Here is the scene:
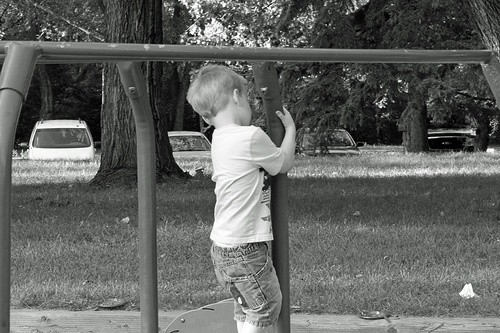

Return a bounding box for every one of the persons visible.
[186,63,296,332]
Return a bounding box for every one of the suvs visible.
[19,118,96,162]
[296,127,364,158]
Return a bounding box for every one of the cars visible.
[426,126,476,153]
[167,130,213,178]
